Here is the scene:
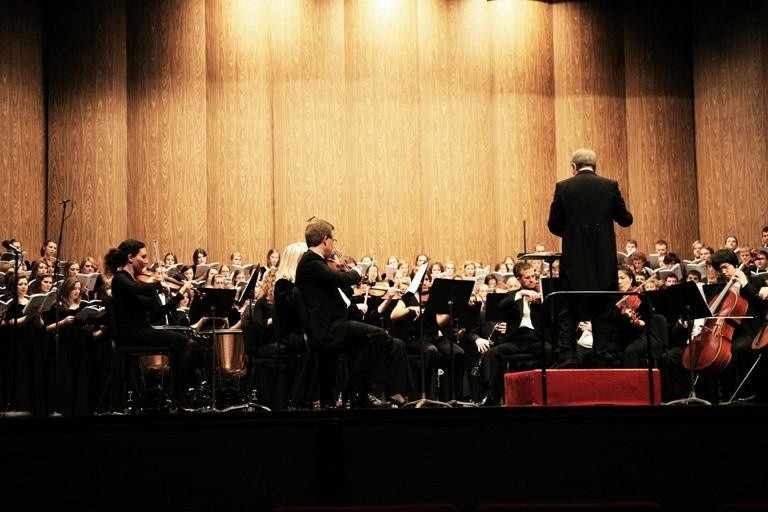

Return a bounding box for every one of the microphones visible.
[57,199,70,204]
[1,240,22,254]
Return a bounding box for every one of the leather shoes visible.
[737,395,760,403]
[477,397,492,406]
[368,393,391,408]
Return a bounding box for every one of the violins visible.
[137,266,201,296]
[352,281,405,297]
[615,274,640,325]
[327,259,375,285]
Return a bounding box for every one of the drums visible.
[216,329,247,378]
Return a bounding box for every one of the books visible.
[644,266,666,274]
[0,260,15,267]
[687,260,707,279]
[191,281,206,287]
[475,266,490,276]
[166,263,183,272]
[0,287,6,290]
[75,273,99,291]
[194,262,220,277]
[75,306,105,321]
[1,298,14,313]
[26,278,37,285]
[0,293,7,298]
[53,280,65,291]
[659,263,683,281]
[617,248,636,264]
[21,292,57,315]
[236,281,249,287]
[648,254,660,268]
[79,299,102,307]
[463,276,485,294]
[357,261,375,273]
[0,272,7,275]
[682,258,702,273]
[231,264,253,271]
[386,265,397,278]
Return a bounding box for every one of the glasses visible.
[331,238,337,245]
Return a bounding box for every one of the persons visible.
[232,270,246,286]
[32,273,54,294]
[724,235,741,264]
[257,247,280,282]
[691,240,705,260]
[201,267,218,287]
[162,252,177,267]
[430,262,443,274]
[463,261,476,277]
[453,275,463,280]
[365,264,381,282]
[189,248,210,281]
[8,239,23,254]
[631,252,654,276]
[62,259,80,279]
[702,249,759,403]
[534,242,545,254]
[484,275,498,288]
[228,252,242,271]
[628,265,636,274]
[758,286,768,300]
[28,260,48,281]
[409,254,428,281]
[617,266,655,369]
[46,277,107,419]
[686,270,701,283]
[547,148,633,369]
[506,276,521,292]
[739,244,752,265]
[469,284,512,406]
[761,226,768,248]
[482,262,561,406]
[38,240,60,261]
[347,287,408,409]
[390,268,466,406]
[172,273,188,281]
[211,274,225,289]
[656,240,668,267]
[634,273,645,286]
[445,261,456,276]
[392,270,403,283]
[360,255,372,263]
[664,274,678,287]
[254,269,279,329]
[495,263,509,273]
[700,257,726,284]
[398,261,408,276]
[504,256,515,273]
[527,259,548,278]
[219,264,231,277]
[254,280,260,301]
[626,239,637,253]
[754,251,768,269]
[104,238,194,412]
[663,252,680,270]
[43,256,55,273]
[182,266,194,280]
[0,275,45,412]
[700,247,715,283]
[177,291,191,309]
[274,243,309,335]
[295,219,394,407]
[80,256,105,300]
[644,279,659,291]
[381,256,399,287]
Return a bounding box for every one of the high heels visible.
[386,395,407,407]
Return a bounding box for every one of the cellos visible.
[682,249,759,402]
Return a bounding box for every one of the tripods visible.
[200,314,222,414]
[661,376,713,405]
[222,305,272,412]
[402,288,452,410]
[442,318,480,409]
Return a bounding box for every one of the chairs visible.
[100,295,176,412]
[288,286,359,412]
